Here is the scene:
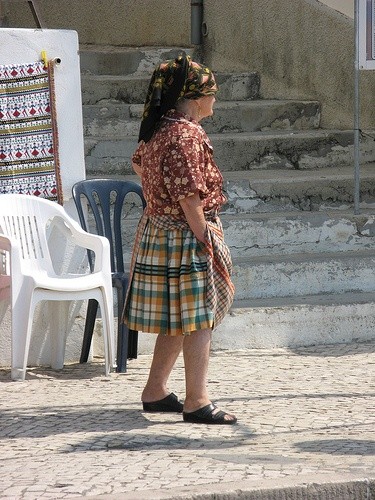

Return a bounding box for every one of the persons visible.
[120,56,238,425]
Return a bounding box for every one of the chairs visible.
[0,193,116,382]
[71,178,146,363]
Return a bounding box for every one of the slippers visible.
[182,400,238,426]
[141,392,185,415]
